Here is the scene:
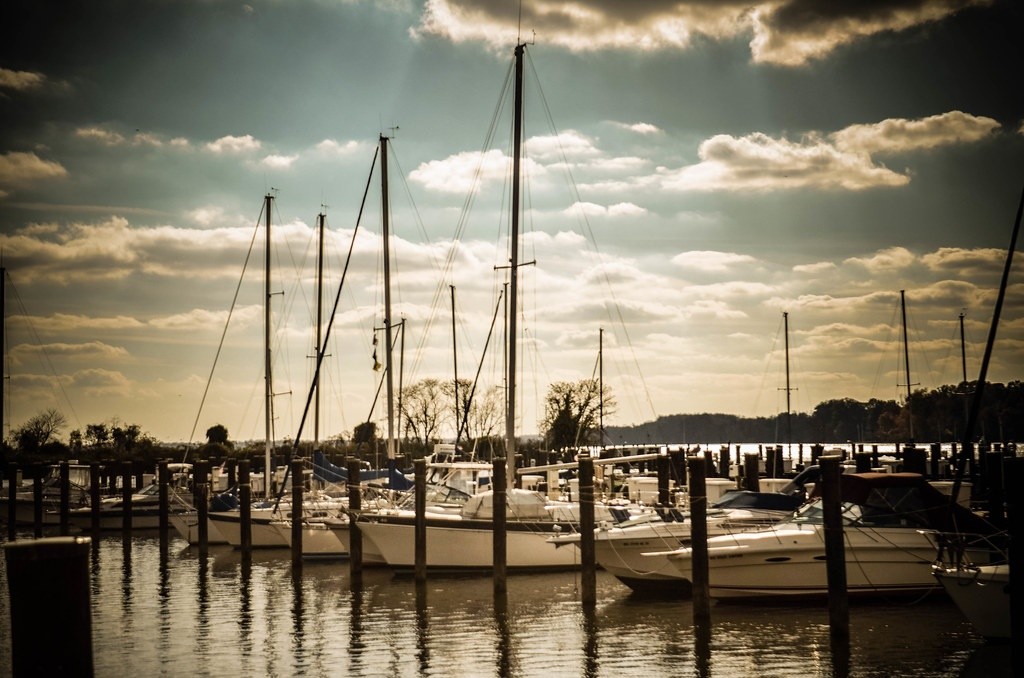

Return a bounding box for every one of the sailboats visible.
[0,0,1024,598]
